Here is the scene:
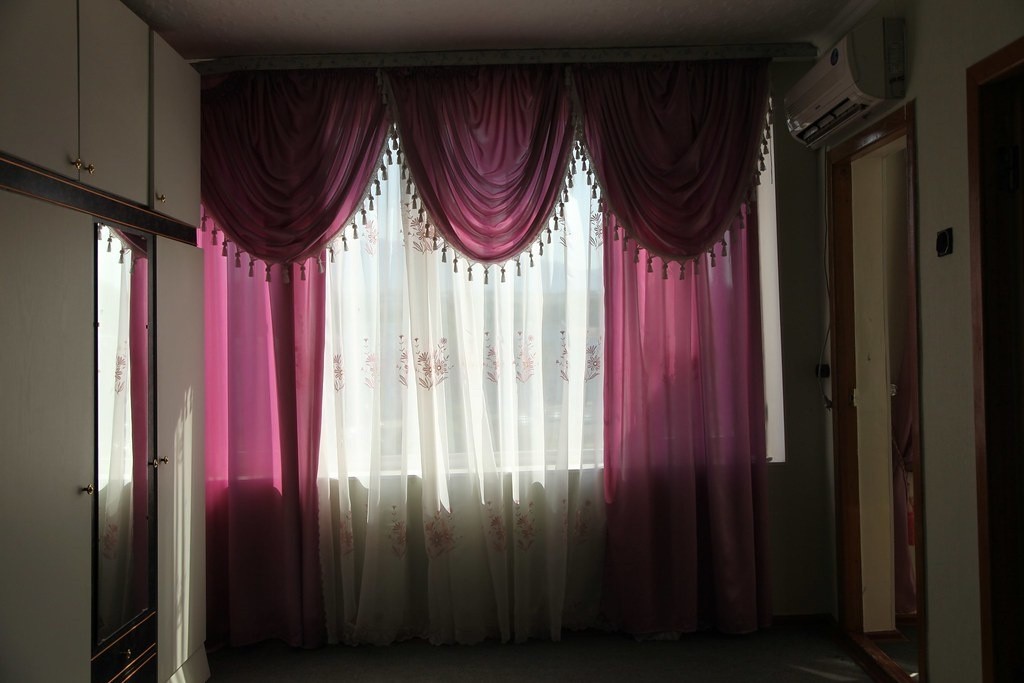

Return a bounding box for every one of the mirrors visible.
[92,221,158,646]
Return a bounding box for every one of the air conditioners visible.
[782,17,909,150]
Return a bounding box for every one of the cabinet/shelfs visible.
[90,609,159,683]
[147,29,203,228]
[0,185,93,682]
[0,0,148,205]
[151,230,206,681]
